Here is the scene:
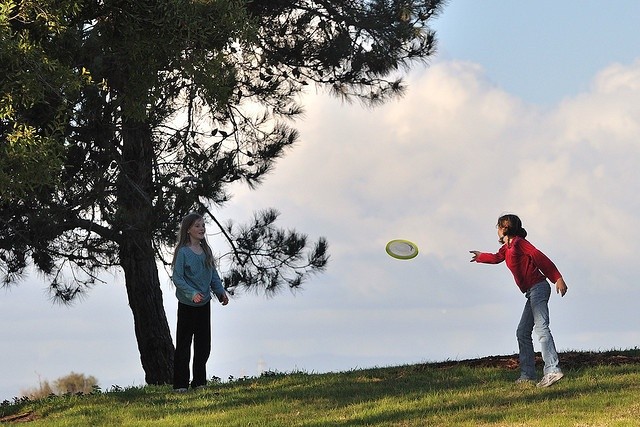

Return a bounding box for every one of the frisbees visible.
[386,239,419,259]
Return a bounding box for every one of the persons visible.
[170,214,229,394]
[469,214,568,388]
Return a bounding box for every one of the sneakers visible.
[514,378,529,383]
[536,368,564,387]
